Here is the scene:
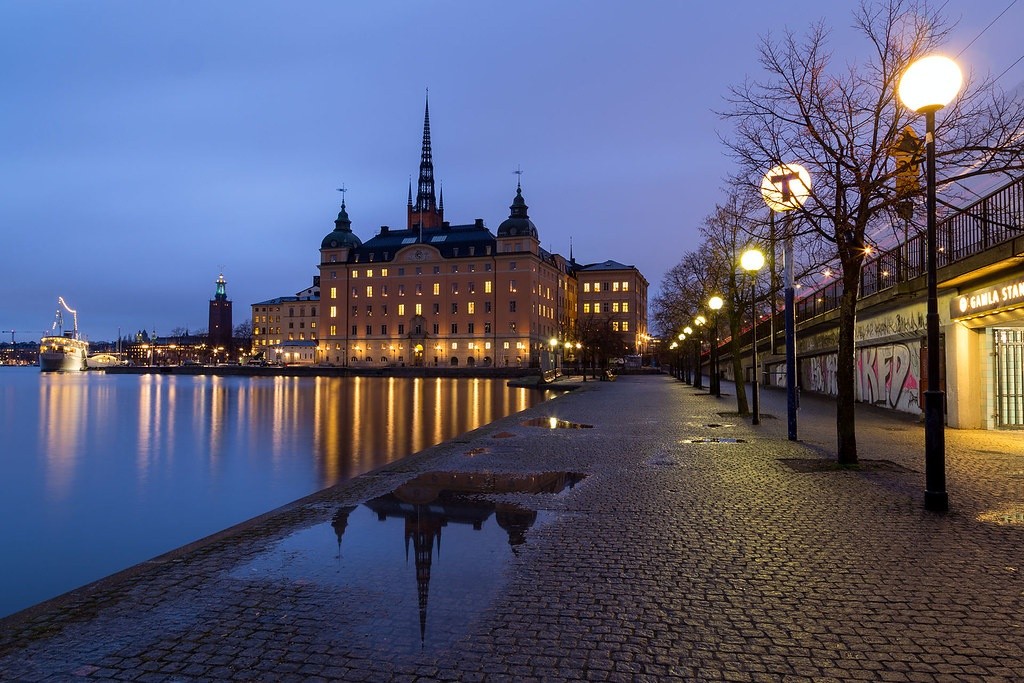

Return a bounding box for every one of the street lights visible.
[684,327,692,385]
[565,343,571,378]
[679,334,686,382]
[740,249,765,425]
[709,296,724,398]
[897,55,964,514]
[551,339,557,382]
[760,163,811,440]
[695,316,706,390]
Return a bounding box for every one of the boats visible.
[86,354,129,370]
[39,295,90,372]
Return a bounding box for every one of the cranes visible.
[1,329,45,346]
